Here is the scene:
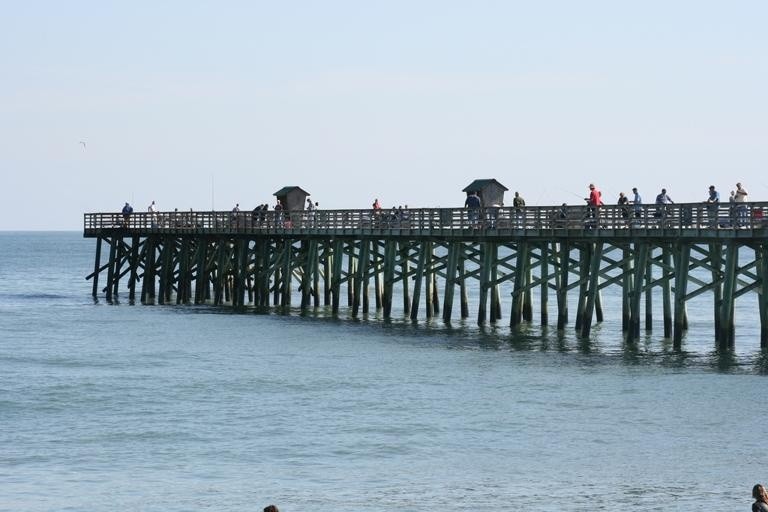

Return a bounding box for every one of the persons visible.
[752,484,768,512]
[463,182,748,229]
[372,198,410,227]
[121,198,320,228]
